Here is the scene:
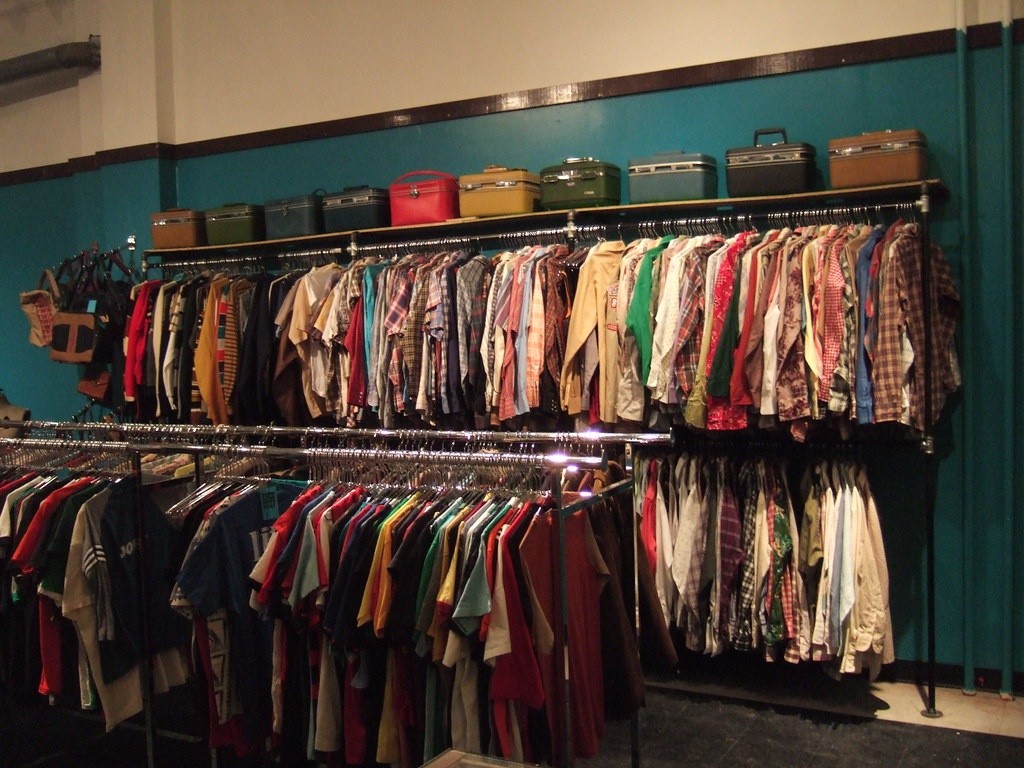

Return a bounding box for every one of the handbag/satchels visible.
[48,265,100,363]
[826,128,928,189]
[50,241,142,346]
[265,196,321,240]
[389,170,458,226]
[78,366,142,405]
[19,270,63,347]
[151,208,207,249]
[204,204,265,245]
[458,164,540,217]
[626,150,732,206]
[724,128,829,203]
[320,184,391,230]
[538,158,623,210]
